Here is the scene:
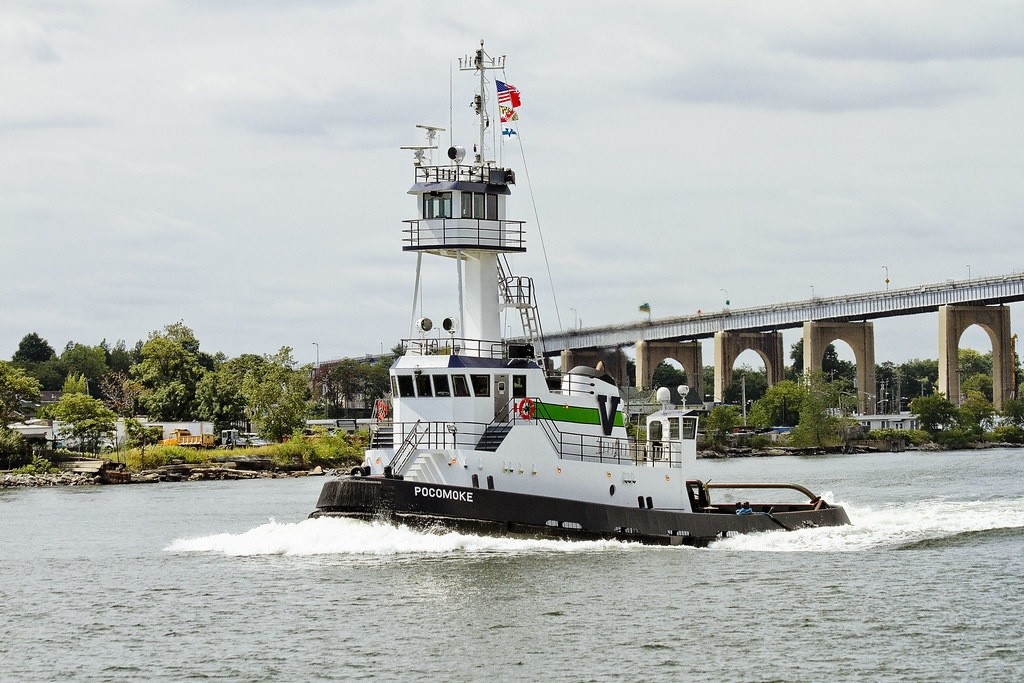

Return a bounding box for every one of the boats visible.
[307,39,853,551]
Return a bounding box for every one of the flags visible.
[640,304,649,311]
[496,79,518,92]
[499,105,518,122]
[501,125,518,138]
[497,91,521,108]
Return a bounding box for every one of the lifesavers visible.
[377,401,388,420]
[519,398,535,420]
